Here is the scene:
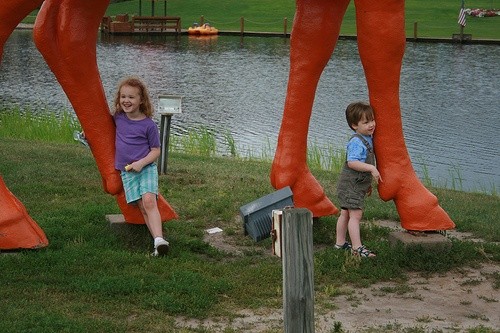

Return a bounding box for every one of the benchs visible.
[131,15,181,32]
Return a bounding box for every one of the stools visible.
[101,13,129,28]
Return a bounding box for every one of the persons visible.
[81,78,170,256]
[335,101,383,257]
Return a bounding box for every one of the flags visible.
[457,0,466,27]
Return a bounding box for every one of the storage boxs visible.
[110,22,134,33]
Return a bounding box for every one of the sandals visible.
[334,242,351,251]
[351,245,376,258]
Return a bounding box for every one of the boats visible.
[188,23,219,35]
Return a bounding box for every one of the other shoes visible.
[154,237,169,256]
